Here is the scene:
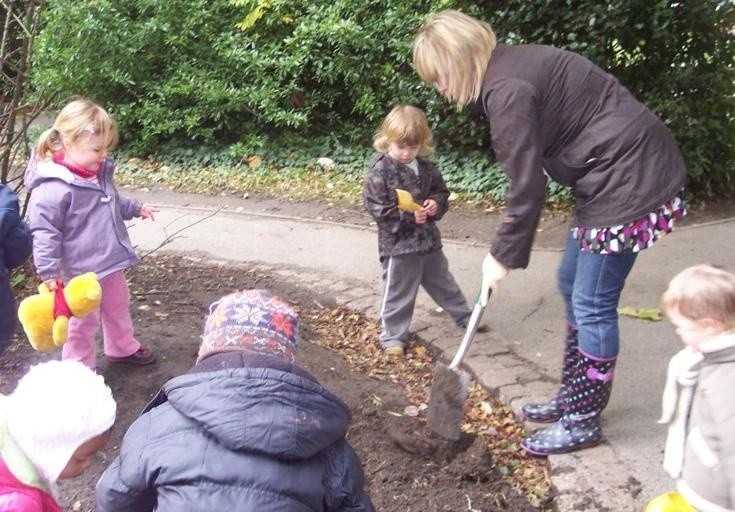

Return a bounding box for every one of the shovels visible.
[427,288,492,442]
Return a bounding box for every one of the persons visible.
[0,171,30,370]
[92,287,374,511]
[411,7,693,457]
[19,96,161,369]
[360,101,487,357]
[657,264,734,511]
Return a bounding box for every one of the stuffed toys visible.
[15,271,103,354]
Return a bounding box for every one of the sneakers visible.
[106,346,156,366]
[460,314,487,330]
[384,346,403,356]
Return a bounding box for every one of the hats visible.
[196,290,301,364]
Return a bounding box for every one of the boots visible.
[520,326,617,455]
